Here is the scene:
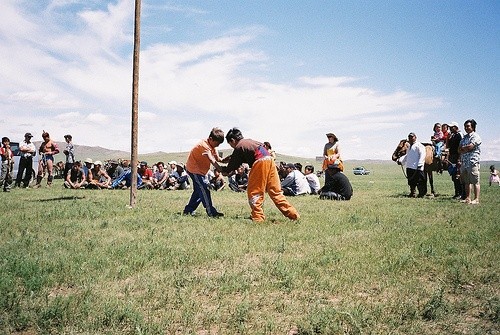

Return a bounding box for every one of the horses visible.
[392,139,450,196]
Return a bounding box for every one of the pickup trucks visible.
[354,165,370,175]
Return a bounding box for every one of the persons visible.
[220,128,300,222]
[459,119,482,205]
[16,133,35,188]
[398,131,427,197]
[0,138,13,192]
[64,135,75,183]
[322,132,345,171]
[448,121,467,200]
[37,132,60,186]
[180,128,225,217]
[56,158,322,196]
[441,123,451,150]
[488,165,500,187]
[432,124,444,156]
[318,164,353,201]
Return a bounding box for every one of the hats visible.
[75,128,343,172]
[448,122,458,128]
[408,132,416,137]
[25,133,33,137]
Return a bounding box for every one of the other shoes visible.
[208,212,224,218]
[14,183,21,189]
[404,189,481,205]
[183,211,200,216]
[64,179,341,200]
[245,216,265,222]
[33,181,42,190]
[23,184,29,188]
[4,186,10,192]
[45,181,52,188]
